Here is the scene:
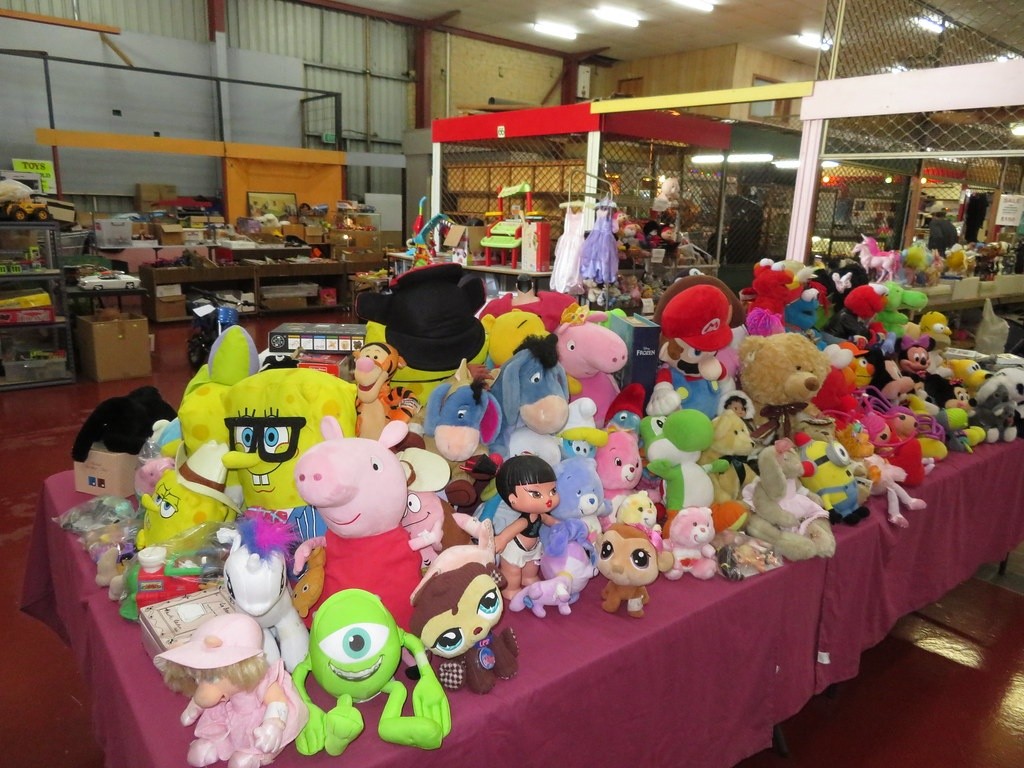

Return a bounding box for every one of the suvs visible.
[3,194,49,222]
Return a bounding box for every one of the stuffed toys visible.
[82,257,1024,768]
[609,211,679,270]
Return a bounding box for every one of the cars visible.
[79,270,141,291]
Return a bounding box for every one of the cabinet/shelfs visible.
[335,210,383,263]
[0,221,76,392]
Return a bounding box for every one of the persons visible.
[475,454,560,599]
[927,203,958,259]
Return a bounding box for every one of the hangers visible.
[559,191,618,210]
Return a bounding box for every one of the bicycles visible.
[184,286,266,366]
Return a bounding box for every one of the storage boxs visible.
[139,588,236,676]
[443,223,500,267]
[72,440,139,499]
[0,184,367,384]
[521,216,551,273]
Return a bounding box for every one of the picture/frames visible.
[247,191,299,225]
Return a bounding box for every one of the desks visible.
[19,431,1024,768]
